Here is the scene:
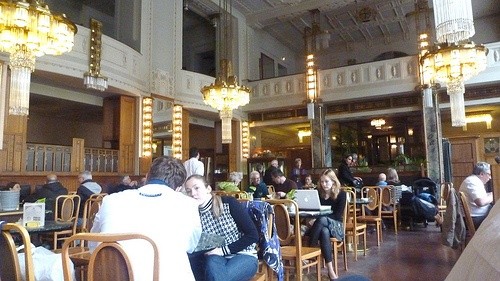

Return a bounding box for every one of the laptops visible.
[294,190,331,211]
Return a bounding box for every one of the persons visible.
[88,148,260,281]
[215,158,315,201]
[0,170,102,247]
[376,168,445,231]
[338,154,357,186]
[301,169,347,281]
[459,162,494,231]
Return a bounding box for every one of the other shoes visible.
[328,273,338,281]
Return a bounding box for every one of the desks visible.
[349,197,372,206]
[288,209,334,236]
[188,234,226,259]
[11,221,71,244]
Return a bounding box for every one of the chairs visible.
[0,181,479,281]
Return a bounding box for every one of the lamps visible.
[242,118,250,158]
[173,103,183,161]
[143,96,153,158]
[0,0,78,116]
[201,0,252,143]
[304,9,332,51]
[417,0,489,127]
[83,19,109,92]
